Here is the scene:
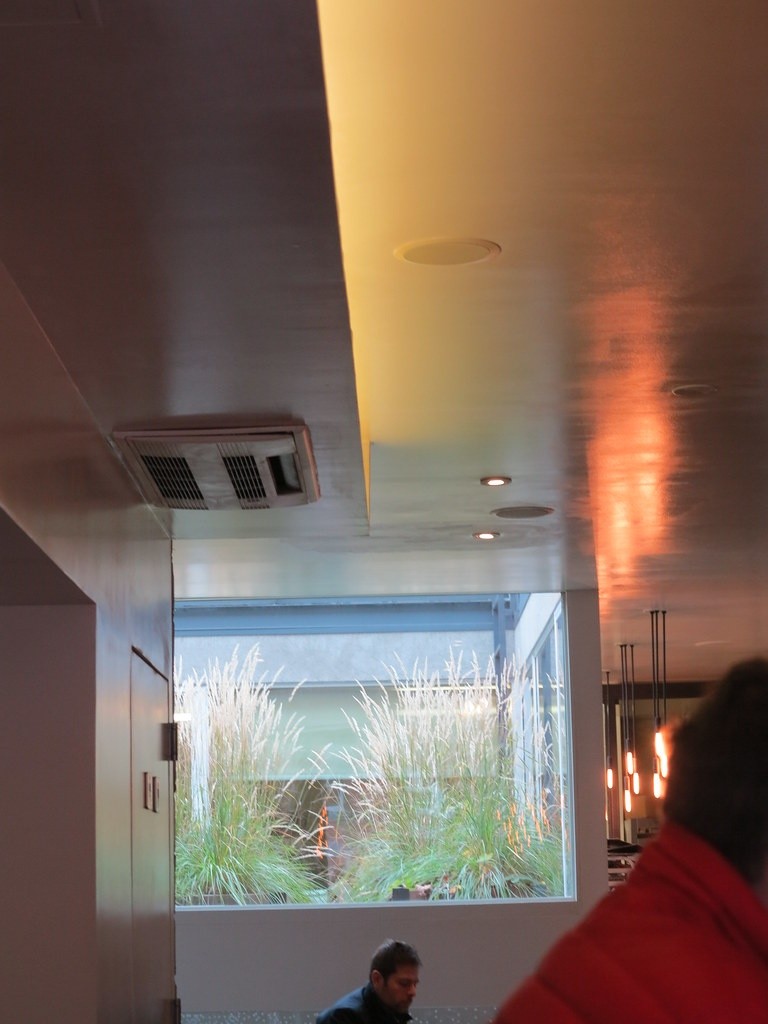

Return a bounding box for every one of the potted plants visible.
[173,643,332,904]
[305,641,569,904]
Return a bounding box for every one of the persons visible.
[491,656,768,1024]
[316,939,423,1024]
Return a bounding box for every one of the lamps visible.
[606,610,668,813]
